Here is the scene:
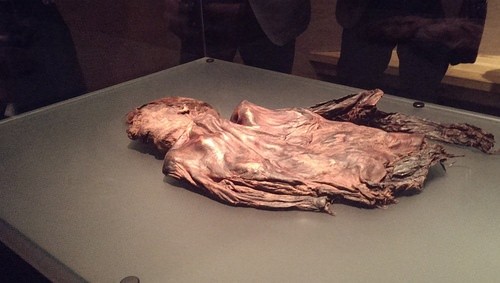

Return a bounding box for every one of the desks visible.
[0,55,500,283]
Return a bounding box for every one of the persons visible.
[0,0,86,125]
[162,1,311,75]
[333,0,490,104]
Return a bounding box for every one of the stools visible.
[309,50,500,116]
